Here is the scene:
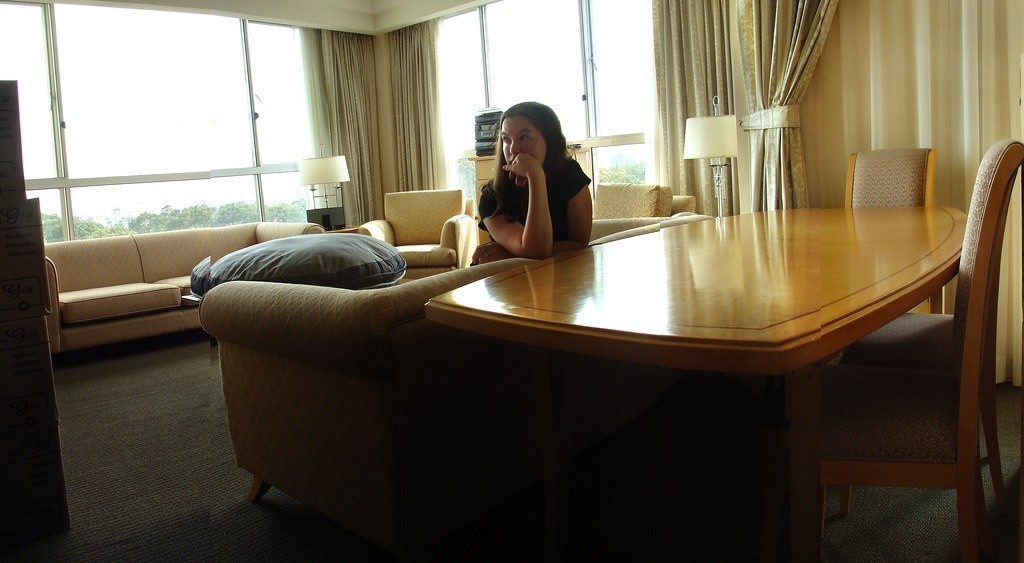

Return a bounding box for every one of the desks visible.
[424,206,966,563]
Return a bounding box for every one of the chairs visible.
[845,148,943,315]
[834,162,1018,520]
[760,138,1024,563]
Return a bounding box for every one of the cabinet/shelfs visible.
[468,155,497,247]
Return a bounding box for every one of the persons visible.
[471,102,593,265]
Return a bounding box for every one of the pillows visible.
[190,234,407,298]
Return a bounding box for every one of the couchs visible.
[359,188,478,280]
[44,221,326,353]
[590,183,697,242]
[203,213,702,524]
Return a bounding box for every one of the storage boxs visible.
[0,80,72,548]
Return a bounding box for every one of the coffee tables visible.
[182,295,218,349]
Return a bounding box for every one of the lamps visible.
[683,115,738,219]
[299,155,350,231]
